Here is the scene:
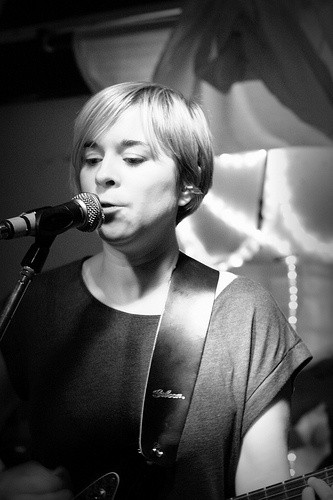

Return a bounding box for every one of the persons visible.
[0,80,333,498]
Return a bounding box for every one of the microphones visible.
[0,192,105,242]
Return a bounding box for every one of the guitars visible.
[73,471,333,500]
